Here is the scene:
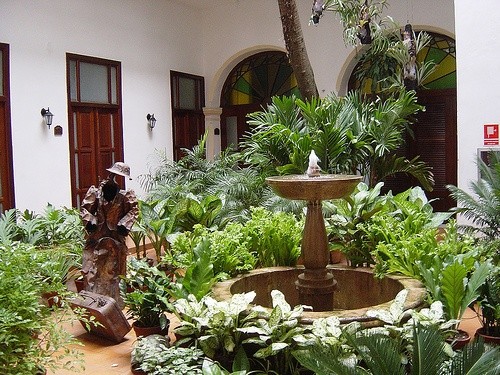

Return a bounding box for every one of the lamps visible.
[147,114,156,131]
[41,107,54,129]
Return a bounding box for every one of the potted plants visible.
[126,200,174,337]
[45,258,88,311]
[296,220,342,263]
[415,251,499,351]
